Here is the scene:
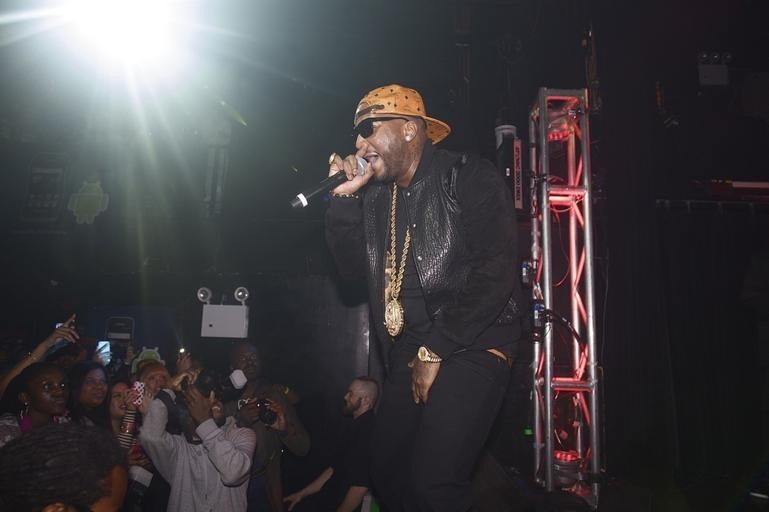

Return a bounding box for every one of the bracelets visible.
[328,190,357,199]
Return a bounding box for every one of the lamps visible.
[195,285,252,339]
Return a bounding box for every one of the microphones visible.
[288,158,368,210]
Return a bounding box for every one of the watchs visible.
[417,345,443,363]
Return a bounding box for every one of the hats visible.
[352,84,453,145]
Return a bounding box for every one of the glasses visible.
[351,116,410,144]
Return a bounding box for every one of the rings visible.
[328,152,336,165]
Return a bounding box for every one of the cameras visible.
[181,369,249,402]
[253,397,277,426]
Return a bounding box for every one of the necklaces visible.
[384,182,411,337]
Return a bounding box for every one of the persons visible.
[323,81,530,512]
